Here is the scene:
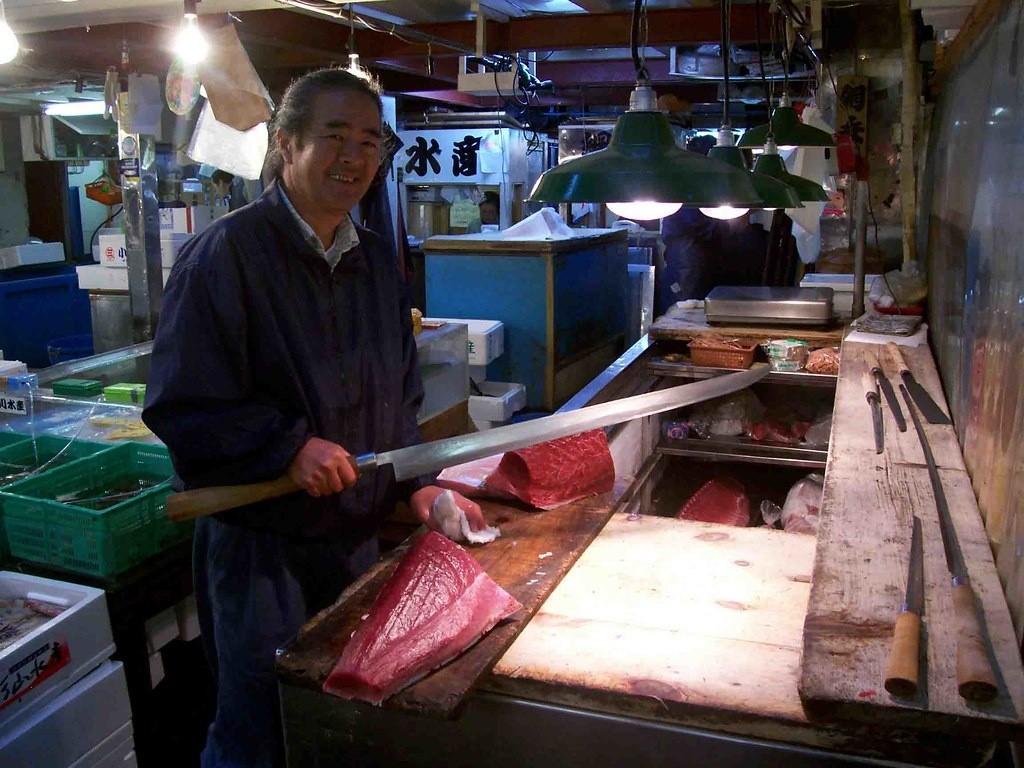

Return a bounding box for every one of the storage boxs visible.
[53,378,102,397]
[0,242,65,270]
[0,431,196,768]
[98,230,194,266]
[0,265,93,367]
[466,381,527,420]
[104,382,146,402]
[75,264,170,290]
[421,315,505,365]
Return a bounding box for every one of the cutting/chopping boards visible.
[274,476,636,720]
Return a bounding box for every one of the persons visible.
[660,133,815,311]
[141,68,487,768]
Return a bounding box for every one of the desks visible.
[423,229,629,412]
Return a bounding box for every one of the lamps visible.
[523,1,837,221]
[40,99,109,119]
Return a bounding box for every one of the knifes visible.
[887,342,953,424]
[167,366,773,521]
[899,385,1000,702]
[865,352,908,431]
[861,375,884,454]
[884,515,927,696]
